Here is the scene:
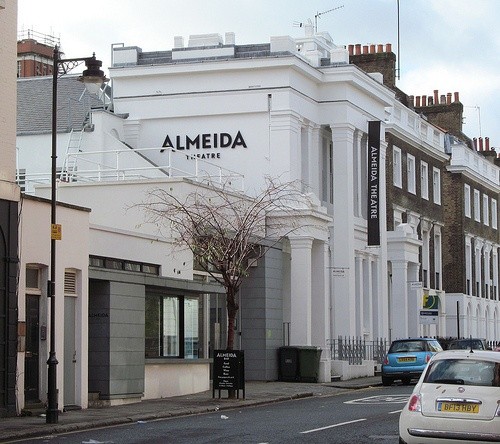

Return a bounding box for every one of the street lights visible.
[46,43,109,423]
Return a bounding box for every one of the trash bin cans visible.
[277,346,323,383]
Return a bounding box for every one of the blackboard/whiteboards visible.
[213,350,246,390]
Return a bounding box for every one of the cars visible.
[382,338,494,386]
[398,349,500,444]
